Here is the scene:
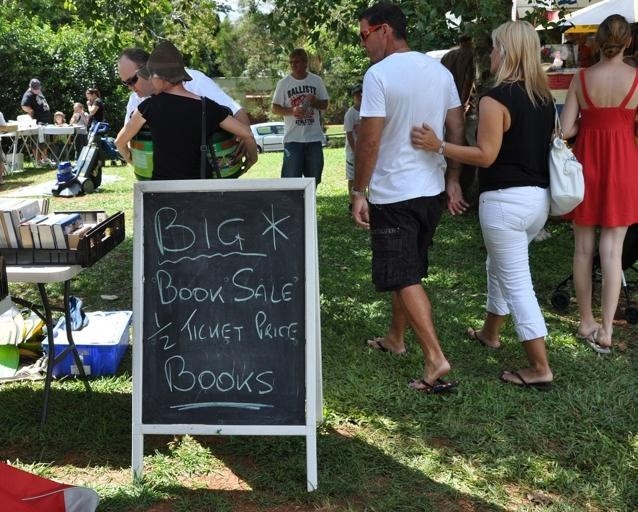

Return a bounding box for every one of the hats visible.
[135,42,192,83]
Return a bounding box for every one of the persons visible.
[561,12,638,349]
[409,18,560,388]
[271,49,329,188]
[347,3,470,393]
[22,77,103,166]
[344,33,495,200]
[111,41,257,180]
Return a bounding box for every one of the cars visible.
[250,121,330,153]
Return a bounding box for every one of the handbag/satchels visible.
[549,138,585,216]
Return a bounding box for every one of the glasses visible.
[358,24,383,41]
[121,74,137,86]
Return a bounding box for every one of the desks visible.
[0,122,89,427]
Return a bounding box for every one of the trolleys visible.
[52,121,112,197]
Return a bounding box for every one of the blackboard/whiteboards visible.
[133,179,317,435]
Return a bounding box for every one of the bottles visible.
[293,98,309,120]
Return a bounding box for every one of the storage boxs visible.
[40,311,133,378]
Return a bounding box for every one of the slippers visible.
[366,338,389,353]
[575,330,609,354]
[464,331,501,350]
[499,372,550,390]
[417,378,458,394]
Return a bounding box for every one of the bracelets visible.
[446,163,461,174]
[349,185,365,198]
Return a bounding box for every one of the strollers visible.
[102,137,129,167]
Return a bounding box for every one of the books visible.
[0,191,107,382]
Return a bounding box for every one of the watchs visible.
[437,136,447,158]
